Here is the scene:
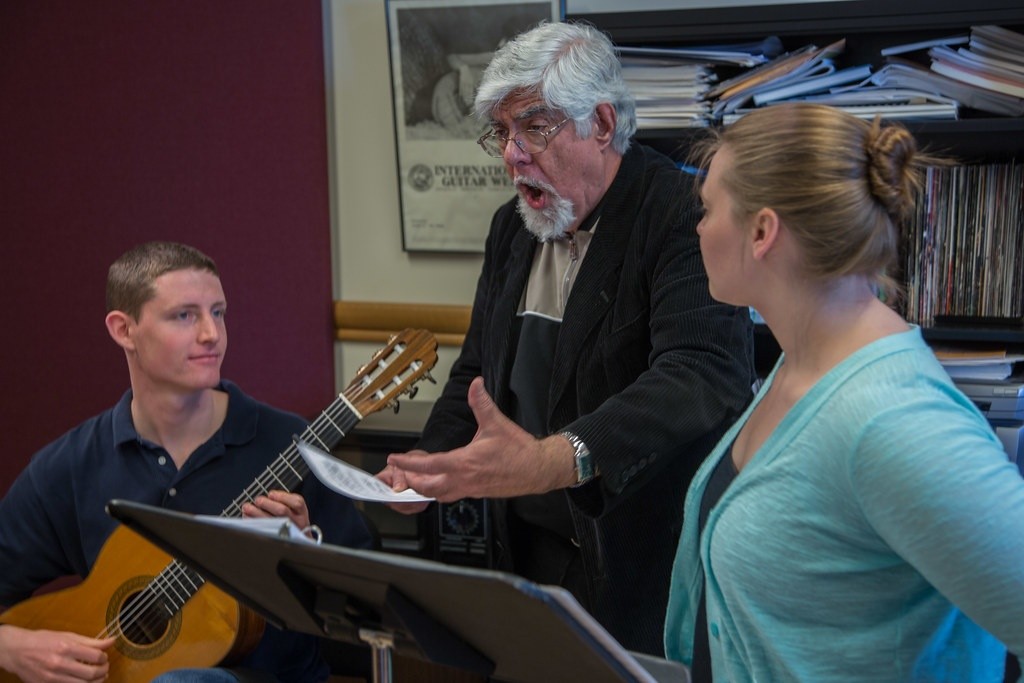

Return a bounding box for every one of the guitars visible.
[0,328,440,683]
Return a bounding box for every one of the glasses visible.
[477,114,574,158]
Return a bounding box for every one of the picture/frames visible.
[384,0,567,256]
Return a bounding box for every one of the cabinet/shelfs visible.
[565,0,1024,478]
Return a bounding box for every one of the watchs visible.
[555,430,596,489]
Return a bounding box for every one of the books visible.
[606,25,1024,381]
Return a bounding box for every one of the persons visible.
[371,18,754,660]
[0,241,383,683]
[662,102,1024,683]
